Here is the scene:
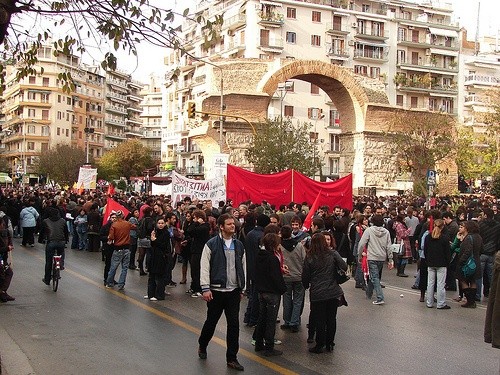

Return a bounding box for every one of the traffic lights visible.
[84,127,94,133]
[188,102,196,119]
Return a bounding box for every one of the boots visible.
[398,264,408,277]
[461,288,476,308]
[420,289,425,302]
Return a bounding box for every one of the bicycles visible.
[37,232,67,292]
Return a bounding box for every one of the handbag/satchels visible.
[461,256,476,279]
[390,238,406,256]
[334,265,349,284]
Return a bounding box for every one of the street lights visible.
[313,113,325,181]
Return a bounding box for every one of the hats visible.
[116,210,124,219]
[109,209,116,218]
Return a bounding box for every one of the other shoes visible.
[144,294,165,301]
[117,284,125,289]
[20,243,35,247]
[179,280,186,284]
[308,345,324,354]
[191,292,203,298]
[185,287,195,293]
[438,305,450,310]
[279,322,298,332]
[105,284,114,289]
[166,281,177,287]
[41,277,50,286]
[326,342,334,354]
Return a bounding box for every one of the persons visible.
[145,219,171,301]
[276,226,305,334]
[185,210,210,298]
[196,215,247,370]
[422,217,453,310]
[466,221,482,300]
[455,221,483,307]
[391,215,413,277]
[136,207,156,275]
[1,178,499,251]
[358,214,394,305]
[175,212,193,283]
[161,212,178,287]
[441,212,460,291]
[251,234,283,356]
[410,210,440,289]
[99,211,121,285]
[242,215,271,327]
[105,211,140,290]
[39,208,70,286]
[477,206,498,302]
[306,231,348,342]
[0,211,15,303]
[300,234,342,353]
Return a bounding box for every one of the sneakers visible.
[197,344,208,359]
[261,348,282,355]
[1,293,15,303]
[229,359,245,372]
[254,342,264,352]
[372,298,384,305]
